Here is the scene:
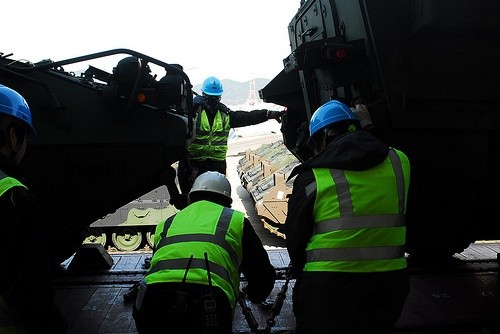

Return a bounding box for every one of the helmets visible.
[1,82,31,124]
[187,170,233,200]
[201,77,224,96]
[307,100,359,148]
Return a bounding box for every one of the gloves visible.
[268,111,286,123]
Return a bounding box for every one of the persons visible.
[136,171,277,334]
[285,101,413,334]
[188,77,285,179]
[0,83,68,334]
